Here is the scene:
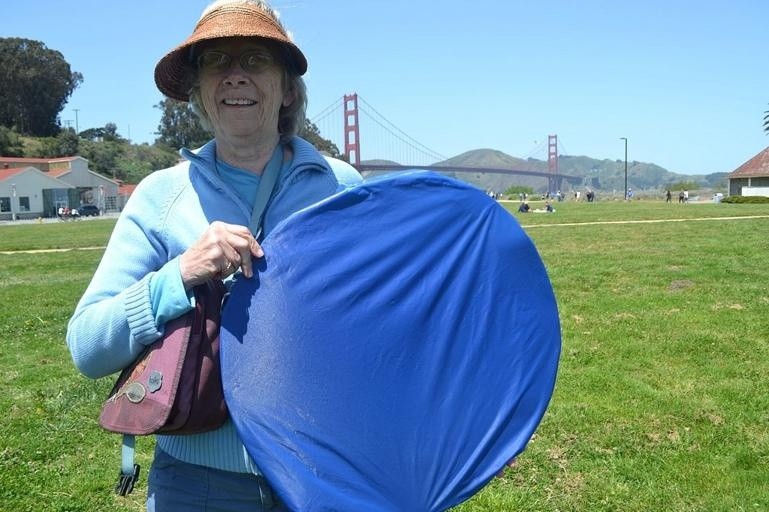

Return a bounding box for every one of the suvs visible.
[74,204,100,217]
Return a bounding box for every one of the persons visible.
[490,188,688,212]
[58,207,78,220]
[66,0,364,512]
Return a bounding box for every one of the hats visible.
[153,3,308,101]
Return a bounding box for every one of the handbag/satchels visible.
[97,281,231,500]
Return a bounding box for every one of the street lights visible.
[620,138,628,200]
[72,108,81,134]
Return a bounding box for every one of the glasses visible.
[195,47,281,75]
